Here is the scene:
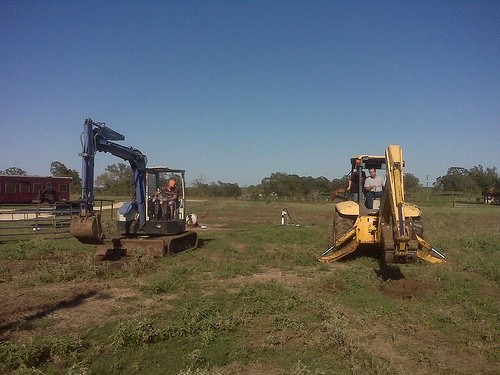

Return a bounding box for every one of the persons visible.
[364,167,385,209]
[153,180,179,221]
[347,168,367,205]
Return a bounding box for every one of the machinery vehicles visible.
[315,145,448,272]
[70,117,199,257]
